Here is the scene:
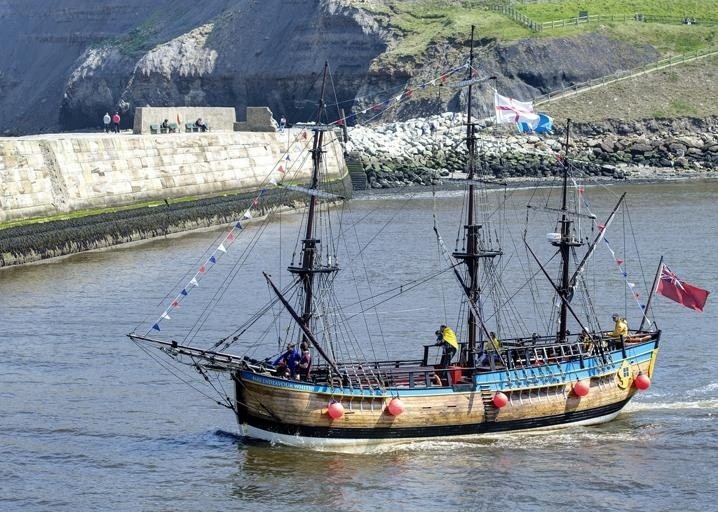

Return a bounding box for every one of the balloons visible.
[389,398,404,415]
[328,403,344,419]
[574,380,589,396]
[494,393,508,408]
[635,375,650,390]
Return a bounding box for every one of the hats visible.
[611,313,618,317]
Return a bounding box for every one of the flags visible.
[495,90,554,134]
[655,263,711,314]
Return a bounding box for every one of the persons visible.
[279,114,287,132]
[435,325,458,366]
[577,327,592,352]
[103,111,120,133]
[272,341,312,382]
[611,314,629,338]
[163,119,176,133]
[195,118,209,132]
[482,332,499,366]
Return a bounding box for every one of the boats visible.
[127,24,661,455]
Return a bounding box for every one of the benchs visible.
[150,123,207,133]
